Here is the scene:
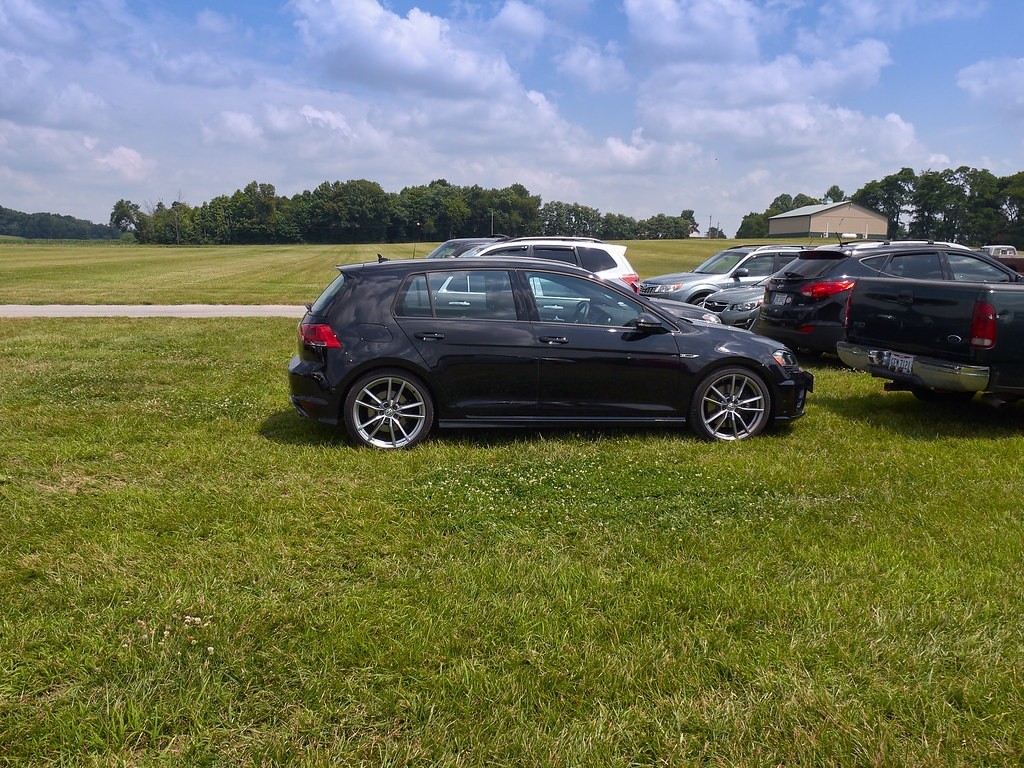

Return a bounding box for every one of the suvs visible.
[703,239,985,331]
[756,245,1007,366]
[441,237,640,299]
[638,244,806,306]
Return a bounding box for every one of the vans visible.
[978,246,1018,256]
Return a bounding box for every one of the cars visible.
[423,237,505,260]
[406,276,722,325]
[287,256,815,451]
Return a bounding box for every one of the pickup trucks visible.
[836,276,1024,413]
